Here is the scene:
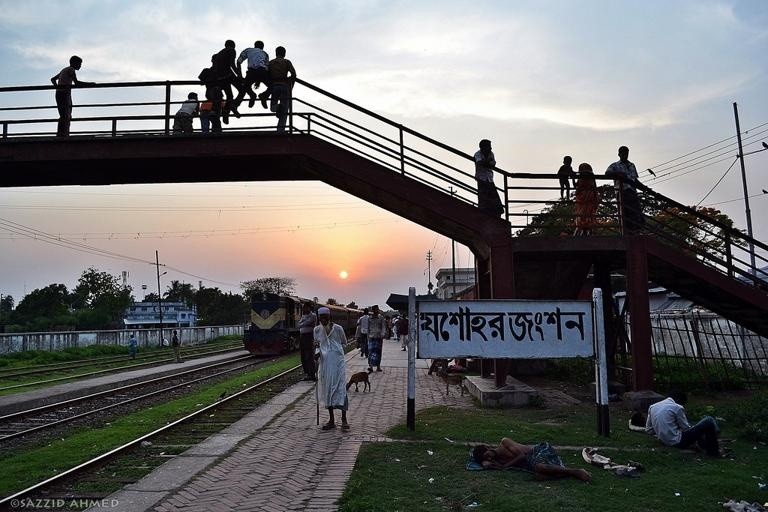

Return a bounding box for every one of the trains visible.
[241,294,379,356]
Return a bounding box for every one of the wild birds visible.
[646,168,656,176]
[761,141,768,149]
[761,189,768,195]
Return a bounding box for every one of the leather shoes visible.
[301,375,317,381]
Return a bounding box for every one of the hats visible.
[318,307,331,316]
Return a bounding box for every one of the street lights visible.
[154,250,170,349]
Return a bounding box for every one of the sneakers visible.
[322,421,351,432]
[575,468,593,484]
[714,438,736,461]
[361,338,408,372]
[222,94,286,123]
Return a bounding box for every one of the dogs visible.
[346,370,372,393]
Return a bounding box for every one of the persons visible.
[313,307,351,432]
[604,146,645,236]
[173,39,296,137]
[51,56,96,137]
[162,339,170,347]
[355,305,408,372]
[297,303,318,381]
[645,390,720,457]
[474,139,504,217]
[557,156,576,199]
[473,437,592,485]
[571,163,598,239]
[172,331,184,363]
[127,335,138,359]
